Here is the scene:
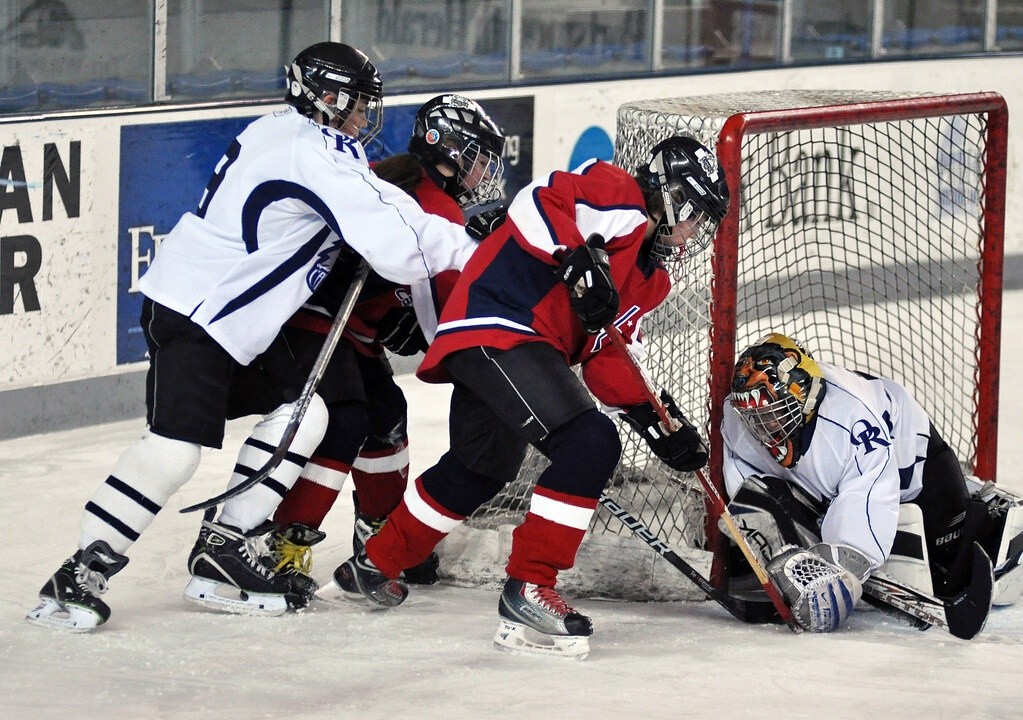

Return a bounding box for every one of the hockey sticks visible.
[558,292,998,644]
[175,236,385,515]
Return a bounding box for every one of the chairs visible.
[0,39,714,117]
[756,27,1023,50]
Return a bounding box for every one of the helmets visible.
[407,94,521,209]
[635,137,731,262]
[285,42,385,146]
[731,333,820,446]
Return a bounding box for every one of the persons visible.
[272,94,505,613]
[23,41,509,633]
[332,136,731,668]
[719,332,1023,642]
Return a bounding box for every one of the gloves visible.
[553,232,619,332]
[375,306,424,356]
[464,205,511,242]
[619,389,708,472]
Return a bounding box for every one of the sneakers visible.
[24,540,127,632]
[183,505,293,617]
[314,548,409,609]
[351,490,440,587]
[266,523,327,612]
[494,577,593,660]
